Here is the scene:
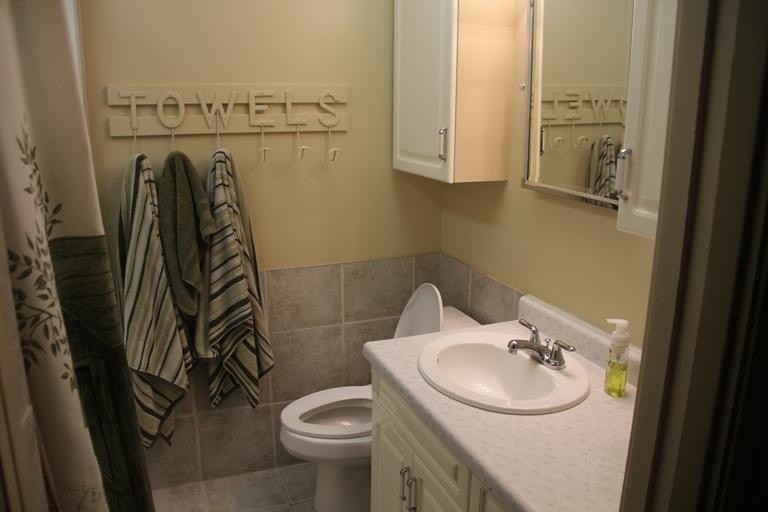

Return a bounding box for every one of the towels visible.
[589,132,621,197]
[197,149,276,406]
[118,149,193,451]
[154,149,209,321]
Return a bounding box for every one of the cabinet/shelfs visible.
[369,366,496,512]
[393,1,531,183]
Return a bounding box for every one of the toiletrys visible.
[604,317,632,399]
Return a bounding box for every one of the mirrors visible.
[524,1,634,206]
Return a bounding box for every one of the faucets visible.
[508,337,549,358]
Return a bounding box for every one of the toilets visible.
[278,282,484,511]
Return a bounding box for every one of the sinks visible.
[418,332,590,412]
